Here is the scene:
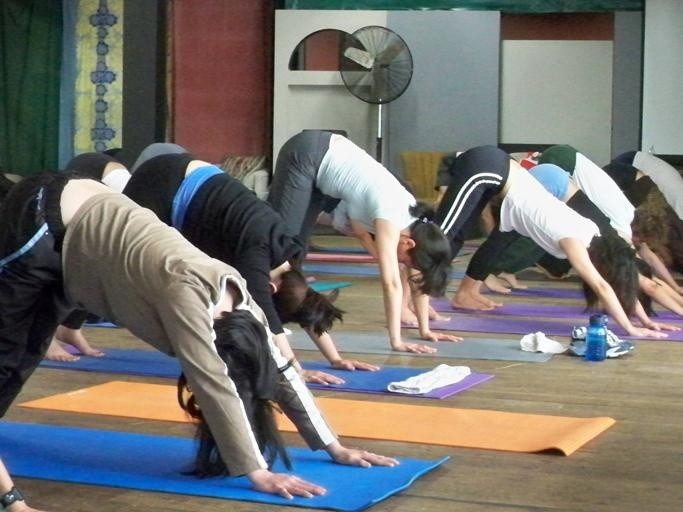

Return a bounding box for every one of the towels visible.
[515,330,568,356]
[387,361,472,396]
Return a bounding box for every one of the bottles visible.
[584,314,608,361]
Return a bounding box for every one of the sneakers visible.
[567,324,637,359]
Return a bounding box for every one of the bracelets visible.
[0,486,23,507]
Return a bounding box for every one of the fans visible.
[338,23,415,164]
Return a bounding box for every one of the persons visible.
[296,187,378,260]
[265,129,463,354]
[401,143,683,342]
[0,170,400,500]
[42,153,382,386]
[65,147,136,192]
[0,459,47,512]
